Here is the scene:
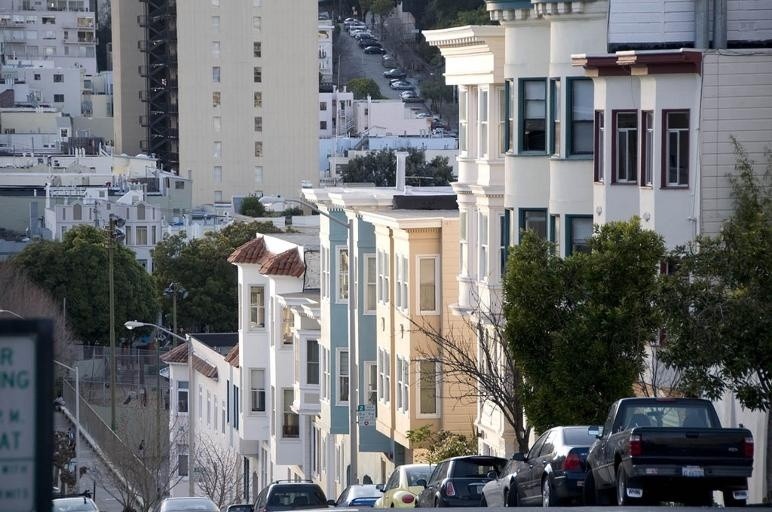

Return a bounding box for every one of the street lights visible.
[125,320,193,496]
[163,280,189,349]
[152,322,166,510]
[259,196,358,485]
[111,212,128,448]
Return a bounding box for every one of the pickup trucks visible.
[581,397,754,506]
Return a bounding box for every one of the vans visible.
[414,455,509,508]
[250,479,328,512]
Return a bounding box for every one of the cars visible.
[327,483,387,507]
[51,496,100,512]
[344,17,457,137]
[508,425,605,507]
[373,463,438,509]
[480,452,529,508]
[151,495,220,511]
[319,14,329,19]
[218,503,255,512]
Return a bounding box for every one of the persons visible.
[72,467,92,498]
[54,391,65,409]
[139,440,145,458]
